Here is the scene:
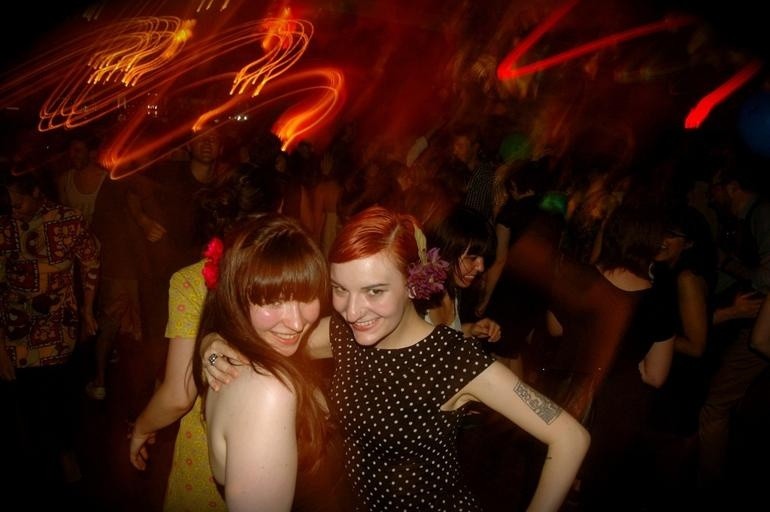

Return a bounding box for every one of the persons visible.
[2,24,770,512]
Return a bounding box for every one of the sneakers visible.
[83,380,106,401]
